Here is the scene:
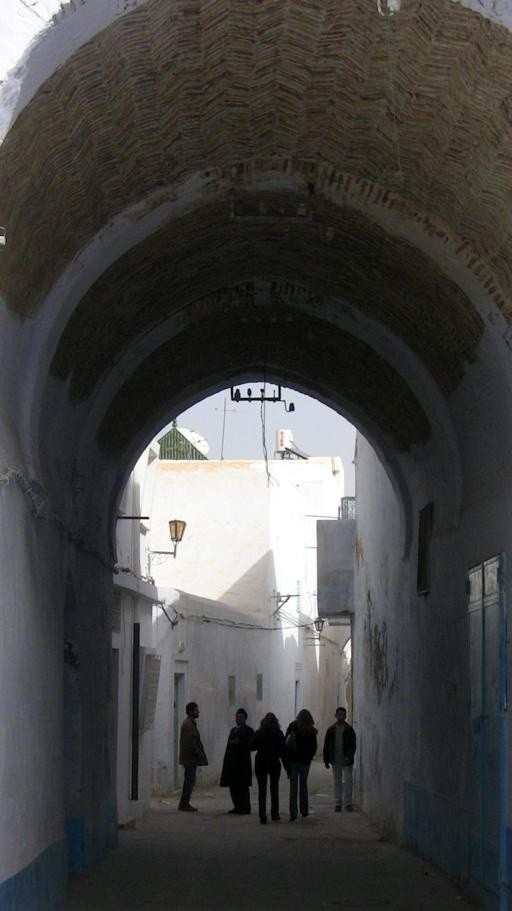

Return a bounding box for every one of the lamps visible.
[144,519,186,579]
[305,615,325,640]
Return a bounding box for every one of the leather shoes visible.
[183,804,197,810]
[229,809,309,825]
[335,805,341,811]
[346,805,353,810]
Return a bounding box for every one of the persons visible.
[323,707,356,812]
[283,709,317,820]
[220,708,255,815]
[177,702,208,811]
[254,712,285,825]
